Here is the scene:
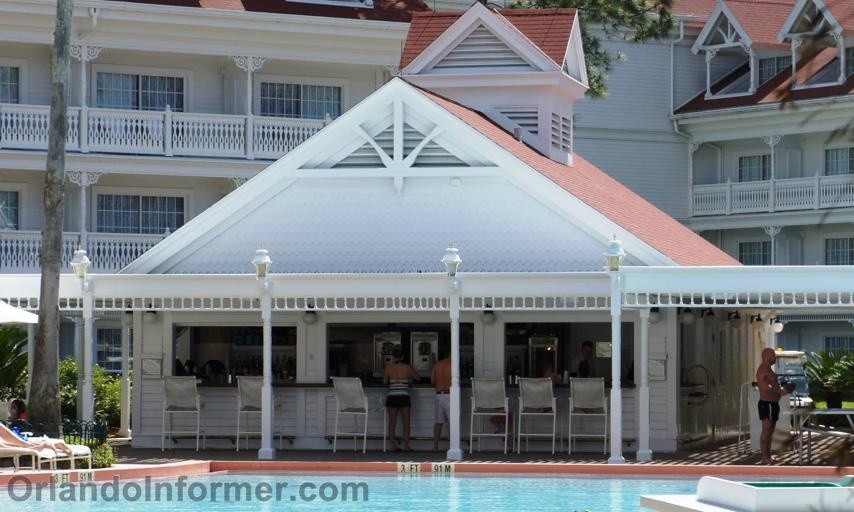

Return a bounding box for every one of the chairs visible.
[159,373,608,458]
[9,427,92,471]
[0,421,54,470]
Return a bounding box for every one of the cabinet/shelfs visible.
[197,340,231,375]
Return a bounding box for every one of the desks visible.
[781,408,854,467]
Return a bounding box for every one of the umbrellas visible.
[0,300,39,325]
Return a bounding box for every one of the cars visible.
[96,344,135,372]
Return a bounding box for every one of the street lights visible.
[71,240,98,434]
[602,230,632,465]
[251,241,278,459]
[440,239,464,463]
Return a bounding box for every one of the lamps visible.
[122,301,132,326]
[303,301,318,326]
[143,302,157,326]
[480,302,497,325]
[649,302,784,334]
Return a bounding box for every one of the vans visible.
[774,349,812,400]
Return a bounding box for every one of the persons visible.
[756,347,790,465]
[538,340,598,385]
[10,400,29,427]
[383,344,420,453]
[430,343,450,452]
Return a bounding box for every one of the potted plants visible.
[801,343,853,409]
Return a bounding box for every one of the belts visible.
[436,390,450,394]
